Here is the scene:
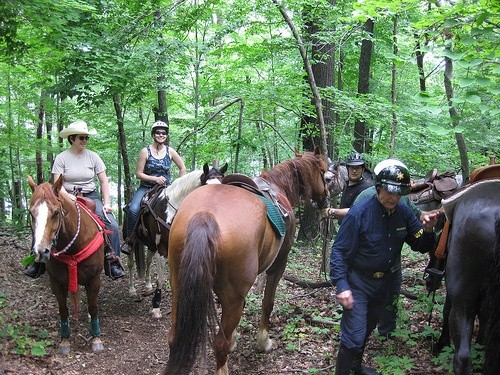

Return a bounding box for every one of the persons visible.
[330,165,437,375]
[122,121,186,254]
[23,121,126,280]
[321,152,373,225]
[353,159,445,340]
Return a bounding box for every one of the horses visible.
[123,163,229,317]
[158,145,327,375]
[28,173,105,356]
[429,177,500,375]
[323,155,464,210]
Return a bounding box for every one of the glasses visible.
[154,130,167,136]
[79,136,90,141]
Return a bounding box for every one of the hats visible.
[60,119,97,137]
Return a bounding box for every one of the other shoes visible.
[104,263,126,279]
[23,264,45,277]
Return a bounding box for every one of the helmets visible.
[151,120,169,131]
[374,166,412,195]
[345,151,365,167]
